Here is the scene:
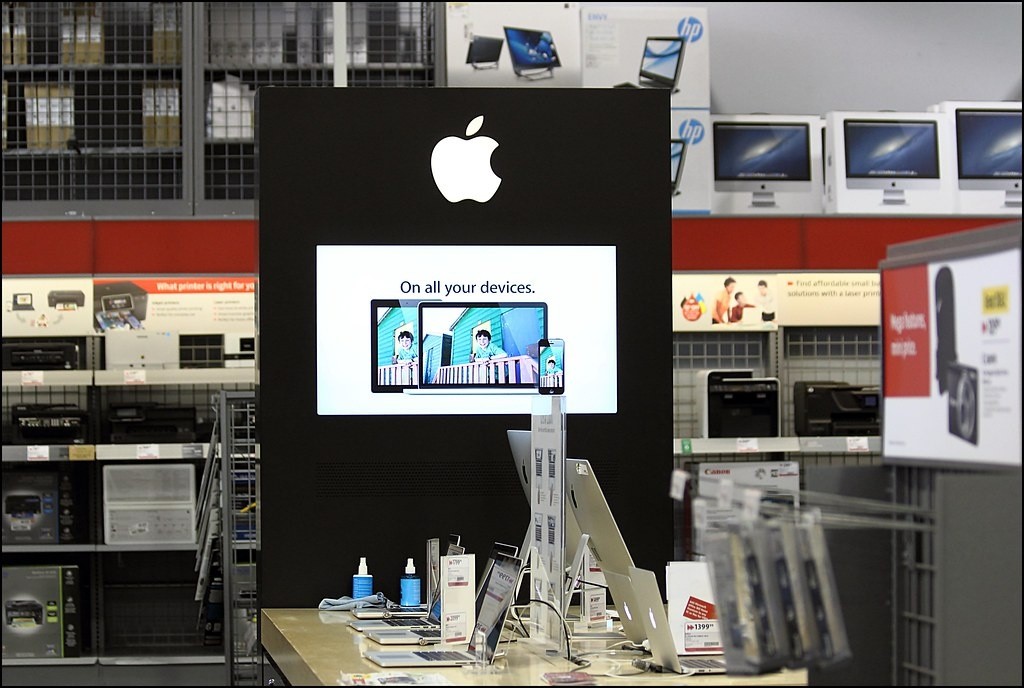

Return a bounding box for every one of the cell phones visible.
[538,339,566,395]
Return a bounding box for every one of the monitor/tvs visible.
[503,26,563,69]
[506,429,583,569]
[955,108,1024,191]
[564,459,634,577]
[670,139,687,195]
[843,118,944,190]
[640,37,686,88]
[466,36,504,64]
[713,122,813,192]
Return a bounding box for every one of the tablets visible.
[372,300,442,394]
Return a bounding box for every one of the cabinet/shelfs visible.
[3,2,1021,688]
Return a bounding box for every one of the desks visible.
[259,603,811,688]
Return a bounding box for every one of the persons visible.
[729,292,757,322]
[473,329,509,384]
[397,330,418,365]
[547,360,562,387]
[712,277,736,325]
[757,280,776,322]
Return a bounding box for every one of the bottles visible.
[352,557,373,599]
[399,558,421,607]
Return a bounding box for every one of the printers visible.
[5,600,43,628]
[794,380,882,435]
[5,495,42,523]
[48,290,84,310]
[93,281,148,334]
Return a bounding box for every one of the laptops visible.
[13,293,35,310]
[362,552,524,668]
[627,565,727,674]
[364,541,518,645]
[351,534,461,619]
[601,571,648,644]
[406,302,548,395]
[347,543,465,631]
